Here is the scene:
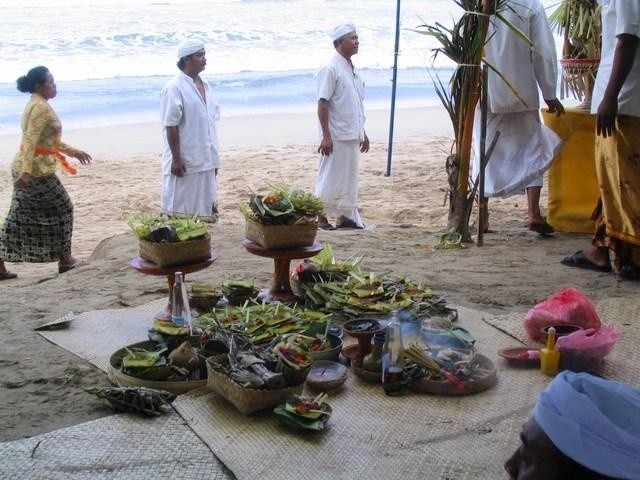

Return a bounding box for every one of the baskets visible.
[206,353,306,415]
[188,291,223,312]
[245,217,317,250]
[139,233,211,268]
[307,334,344,361]
[279,356,312,386]
[226,286,260,306]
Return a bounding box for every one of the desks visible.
[243,239,324,309]
[129,253,219,322]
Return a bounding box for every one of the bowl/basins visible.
[542,324,583,338]
[306,327,345,358]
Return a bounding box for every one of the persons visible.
[504,368,640,480]
[313,22,370,231]
[159,40,221,219]
[0,66,93,278]
[559,0,640,280]
[467,0,567,233]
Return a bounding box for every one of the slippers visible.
[59,259,82,273]
[1,271,18,279]
[336,219,362,229]
[561,250,611,272]
[319,222,334,230]
[529,216,555,234]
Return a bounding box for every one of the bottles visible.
[169,271,192,329]
[381,308,406,399]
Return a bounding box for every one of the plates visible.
[498,344,542,365]
